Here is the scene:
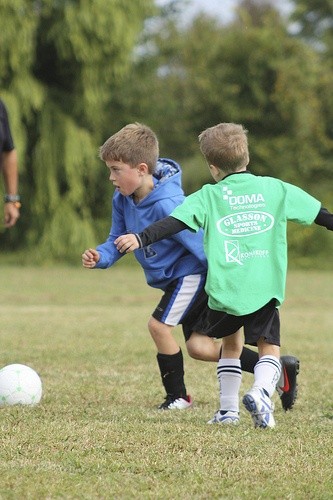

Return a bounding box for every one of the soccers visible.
[0,363,43,409]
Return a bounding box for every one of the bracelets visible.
[3,194,22,210]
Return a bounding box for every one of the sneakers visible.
[244,387,275,431]
[207,410,239,427]
[276,356,300,411]
[157,396,190,411]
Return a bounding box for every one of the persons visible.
[81,121,302,413]
[115,121,333,430]
[1,96,24,227]
